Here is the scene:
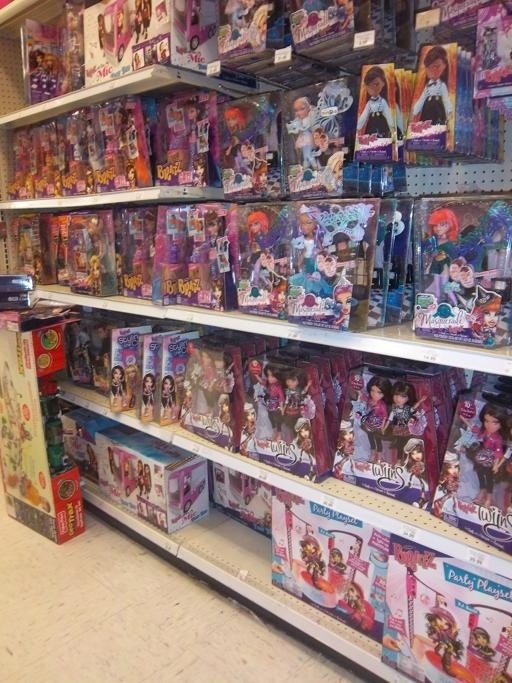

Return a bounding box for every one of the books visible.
[1,273,40,294]
[1,289,42,312]
[1,306,72,333]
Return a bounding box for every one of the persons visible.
[343,581,367,618]
[470,626,497,662]
[424,606,465,677]
[327,547,347,574]
[299,534,325,589]
[8,38,512,556]
[7,38,510,556]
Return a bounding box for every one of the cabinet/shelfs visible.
[0,1,511,683]
